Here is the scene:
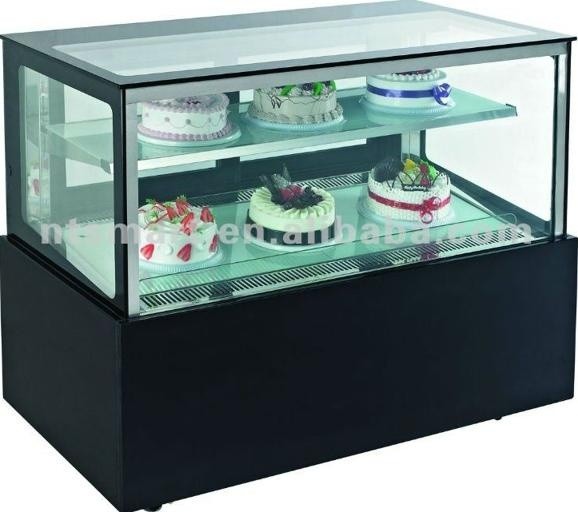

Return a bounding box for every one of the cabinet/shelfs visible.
[0,0,578,512]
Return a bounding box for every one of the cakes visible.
[365,68,452,108]
[245,172,336,246]
[367,155,451,222]
[247,80,344,125]
[137,194,219,265]
[137,93,232,141]
